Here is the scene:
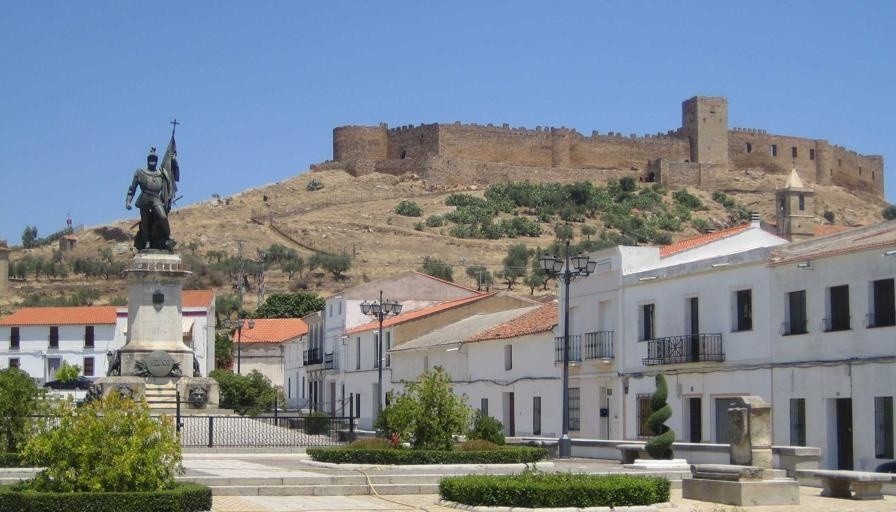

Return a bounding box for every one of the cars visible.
[44,375,94,390]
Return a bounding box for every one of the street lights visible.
[541,238,599,463]
[222,318,255,373]
[357,288,405,424]
